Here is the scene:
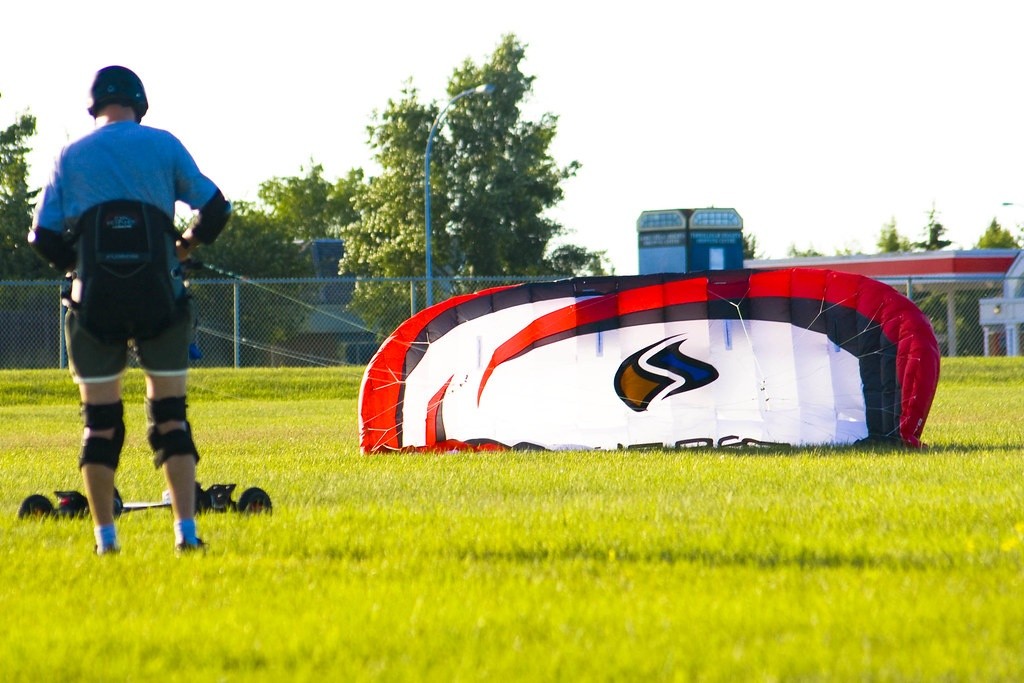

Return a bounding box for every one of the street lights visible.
[423,83,496,309]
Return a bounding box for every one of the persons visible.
[30,66,233,559]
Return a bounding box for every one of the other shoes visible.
[174,537,205,557]
[93,543,120,556]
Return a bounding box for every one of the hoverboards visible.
[18,480,273,519]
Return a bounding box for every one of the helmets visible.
[89,65,149,122]
